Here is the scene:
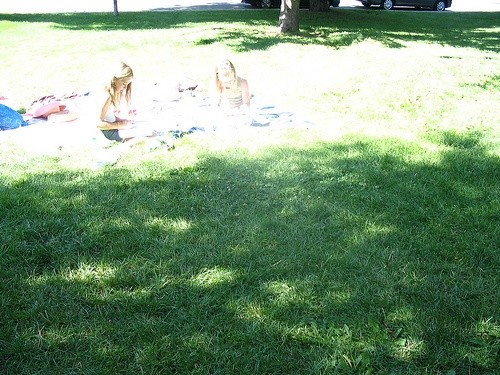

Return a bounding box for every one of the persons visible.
[206,58,254,117]
[31,59,137,132]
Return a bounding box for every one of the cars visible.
[357,0,453,12]
[241,0,341,9]
